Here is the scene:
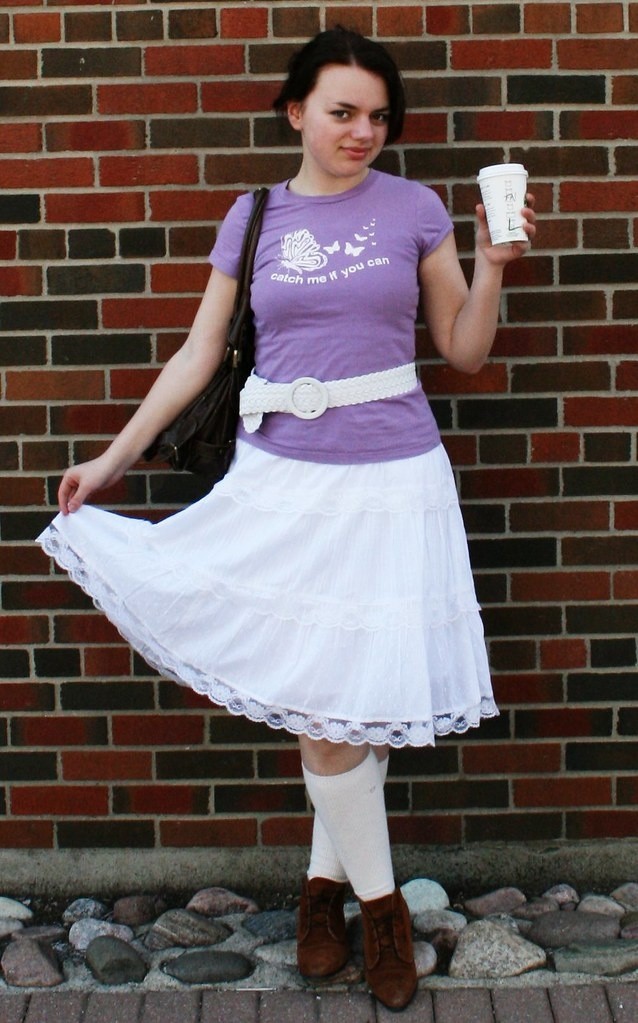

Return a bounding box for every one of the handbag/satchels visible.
[141,187,270,483]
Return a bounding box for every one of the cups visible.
[477,163,528,245]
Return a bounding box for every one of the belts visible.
[238,362,418,434]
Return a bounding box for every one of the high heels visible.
[355,878,418,1012]
[297,876,348,980]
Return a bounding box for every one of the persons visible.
[35,24,535,1012]
